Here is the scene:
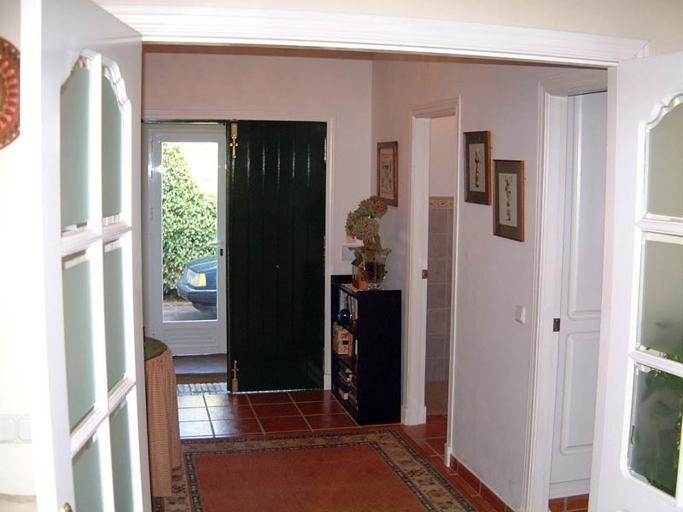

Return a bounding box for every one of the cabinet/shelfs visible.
[330,273,403,425]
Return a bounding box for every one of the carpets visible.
[154,430,476,512]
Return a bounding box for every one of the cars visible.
[175,254,216,318]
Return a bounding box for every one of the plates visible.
[0,35,20,149]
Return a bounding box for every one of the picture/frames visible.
[465,130,492,205]
[375,140,399,207]
[492,159,526,242]
